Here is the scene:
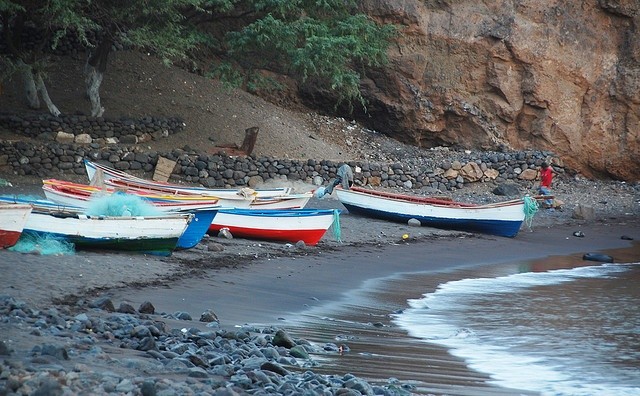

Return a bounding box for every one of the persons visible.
[539,162,554,207]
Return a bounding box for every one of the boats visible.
[250,188,314,210]
[0,194,222,252]
[316,165,555,239]
[84,156,293,198]
[104,176,257,212]
[23,209,195,257]
[42,177,342,248]
[0,202,35,250]
